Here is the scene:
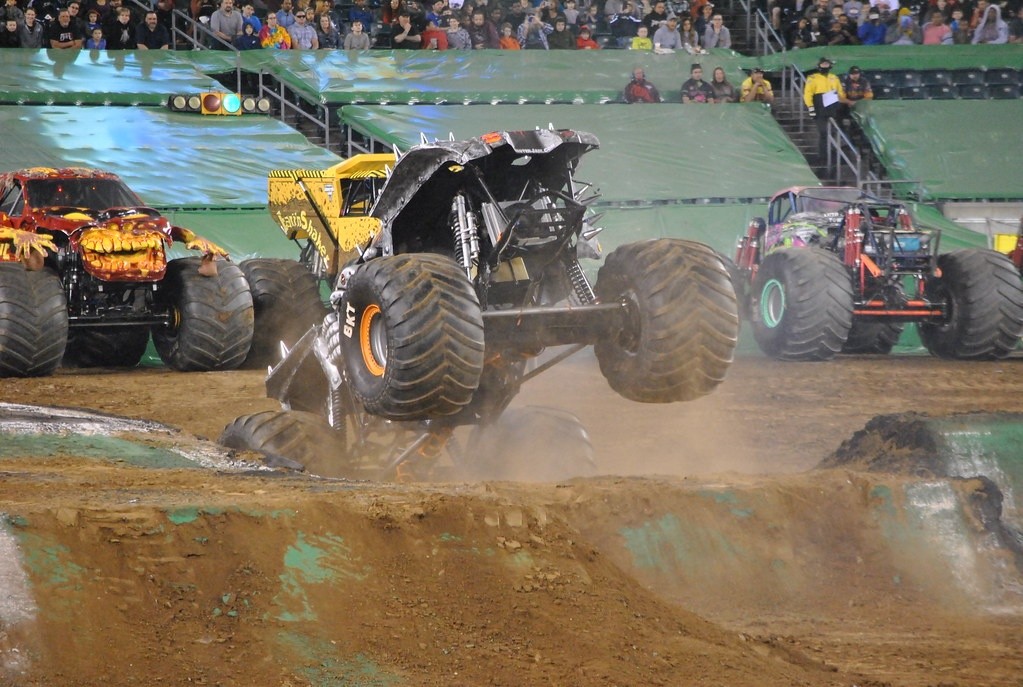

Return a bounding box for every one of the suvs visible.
[733,188,1023,362]
[0,166,256,379]
[236,150,410,364]
[216,124,740,483]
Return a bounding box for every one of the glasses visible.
[295,15,305,18]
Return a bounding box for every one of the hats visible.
[691,64,702,74]
[818,58,831,65]
[666,14,677,21]
[751,68,764,74]
[849,66,860,73]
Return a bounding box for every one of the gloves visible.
[808,107,816,118]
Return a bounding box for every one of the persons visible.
[159,0,375,51]
[681,63,718,105]
[381,0,606,51]
[755,0,1023,52]
[624,66,660,103]
[841,69,874,144]
[805,55,848,159]
[603,0,732,54]
[0,0,169,50]
[740,69,775,103]
[712,67,737,104]
